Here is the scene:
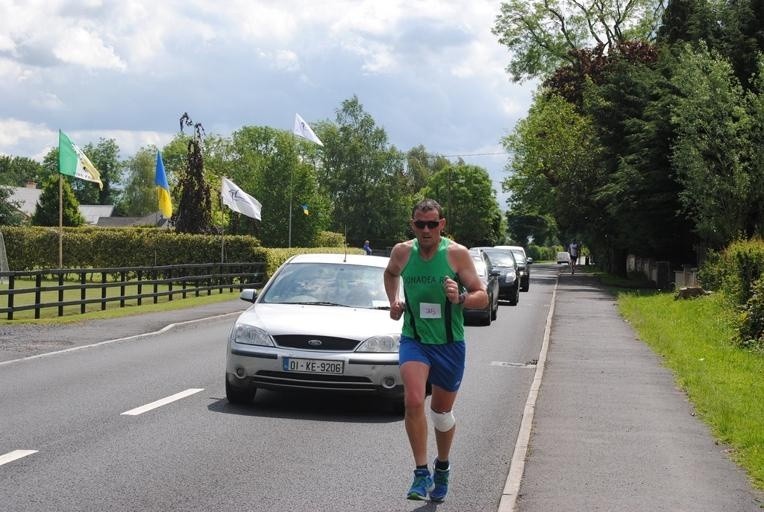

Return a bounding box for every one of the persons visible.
[382,196,490,503]
[567,239,579,275]
[364,239,373,255]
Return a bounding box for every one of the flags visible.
[294,112,325,147]
[59,130,103,192]
[300,204,309,215]
[154,149,173,220]
[221,175,263,223]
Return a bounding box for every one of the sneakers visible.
[407,463,450,502]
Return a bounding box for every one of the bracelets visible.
[458,294,465,305]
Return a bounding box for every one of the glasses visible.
[413,221,439,229]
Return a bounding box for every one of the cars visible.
[223,251,432,416]
[458,245,534,326]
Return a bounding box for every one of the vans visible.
[557,252,571,264]
